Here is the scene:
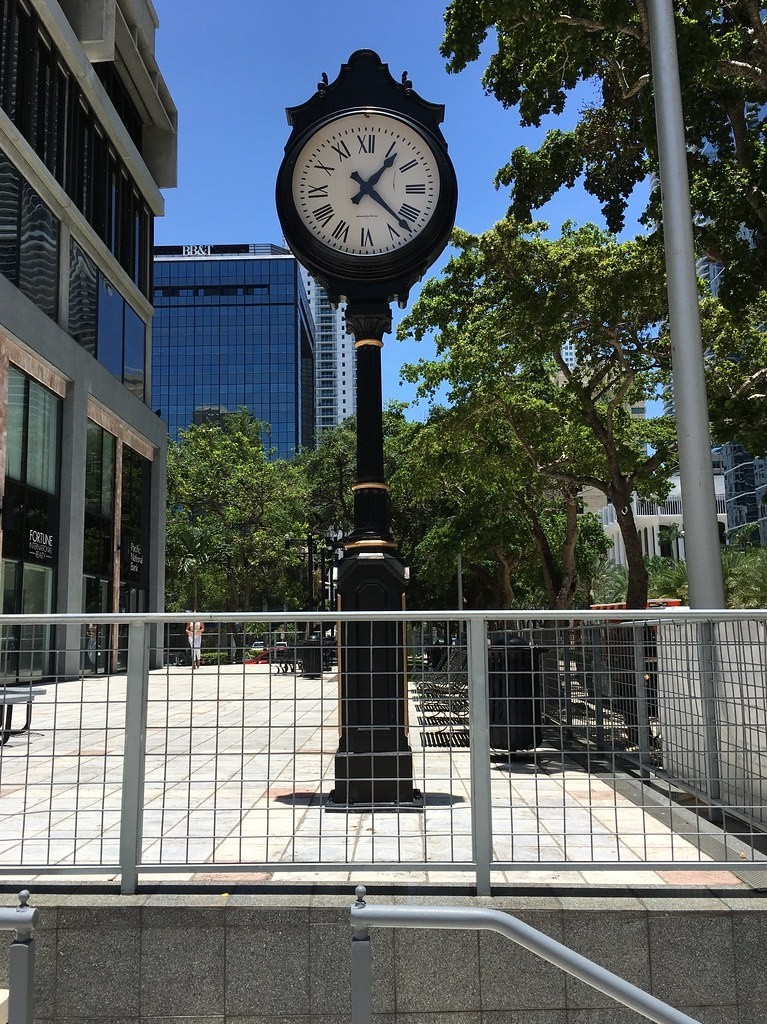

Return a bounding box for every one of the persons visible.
[186,608,205,669]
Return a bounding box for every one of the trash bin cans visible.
[488,634,550,758]
[302,635,321,679]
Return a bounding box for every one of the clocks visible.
[281,101,447,279]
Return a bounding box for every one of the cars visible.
[252,642,266,651]
[163,651,189,666]
[241,647,284,665]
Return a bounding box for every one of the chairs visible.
[272,640,338,676]
[415,647,471,717]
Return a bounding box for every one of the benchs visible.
[0,686,46,743]
